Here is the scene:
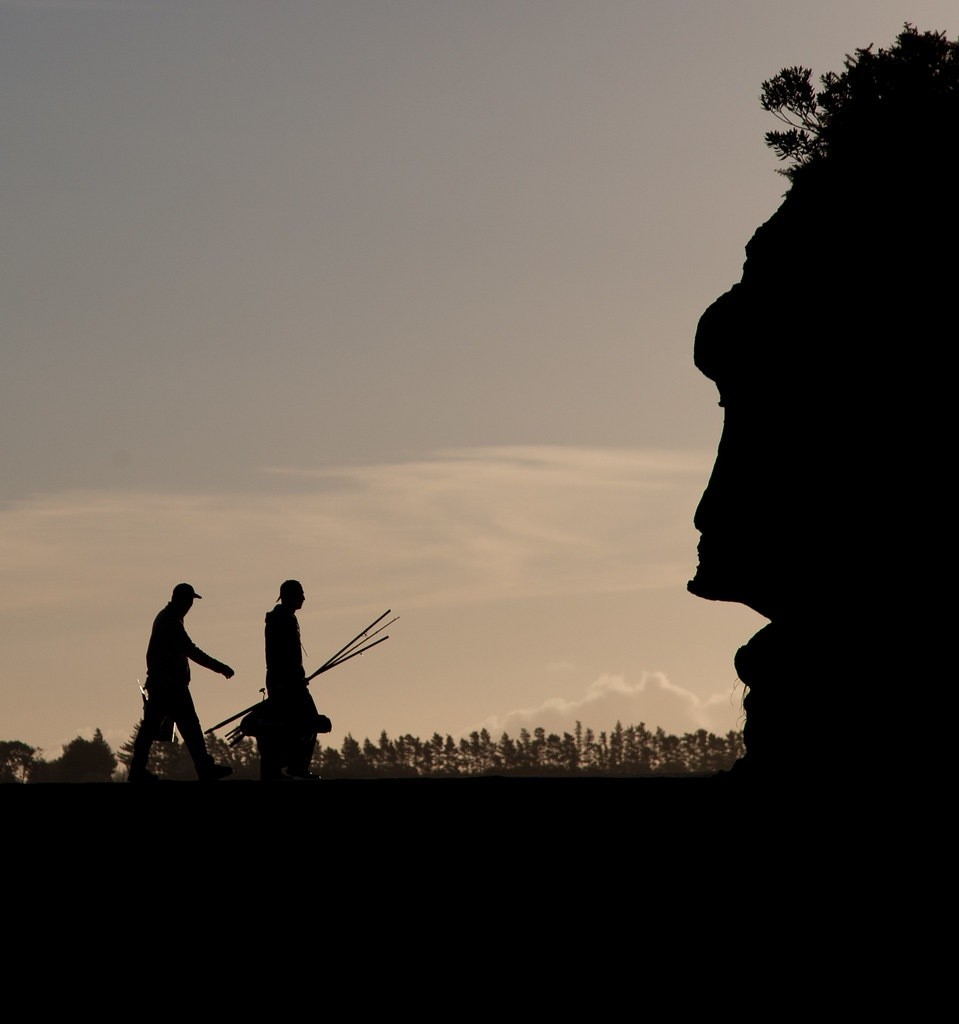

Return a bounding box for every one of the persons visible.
[126,583,234,780]
[260,580,318,777]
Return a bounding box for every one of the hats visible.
[276,580,300,603]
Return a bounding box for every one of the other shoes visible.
[127,768,161,784]
[300,772,322,780]
[199,764,234,781]
